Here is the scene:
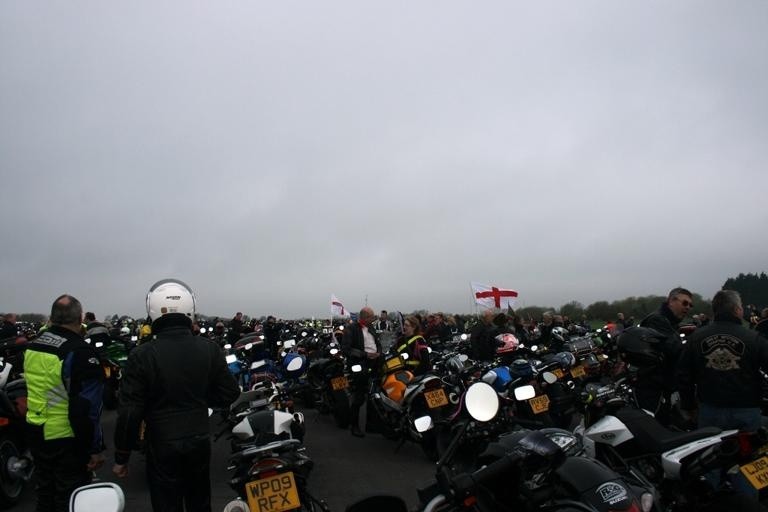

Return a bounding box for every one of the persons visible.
[1,313,284,358]
[680,290,767,434]
[23,295,104,511]
[690,314,706,331]
[112,279,241,511]
[341,307,383,438]
[639,288,693,423]
[330,311,638,356]
[388,315,429,376]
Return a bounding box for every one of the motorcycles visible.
[0,322,768,512]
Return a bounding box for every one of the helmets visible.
[145,278,195,324]
[509,358,532,379]
[555,351,576,368]
[551,326,569,342]
[519,428,586,503]
[495,332,520,353]
